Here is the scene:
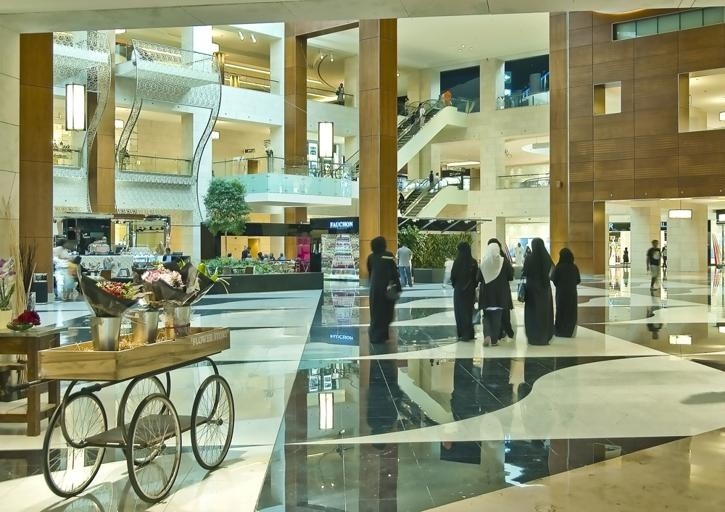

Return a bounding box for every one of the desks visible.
[0,325,67,432]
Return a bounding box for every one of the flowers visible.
[73,264,139,318]
[0,260,16,311]
[134,265,185,306]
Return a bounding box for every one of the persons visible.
[162,247,173,261]
[428,169,435,185]
[435,172,440,184]
[52,239,76,301]
[661,246,668,268]
[365,347,404,450]
[436,342,591,442]
[519,236,556,345]
[441,91,452,106]
[487,238,516,343]
[662,268,668,292]
[336,82,344,105]
[418,101,429,129]
[448,239,479,342]
[622,267,630,288]
[225,246,286,264]
[395,242,414,289]
[397,191,407,214]
[410,111,416,126]
[646,290,666,340]
[366,235,403,345]
[552,246,583,338]
[623,247,629,269]
[443,88,452,106]
[516,243,524,265]
[474,241,517,346]
[524,246,532,257]
[63,229,78,252]
[647,239,662,293]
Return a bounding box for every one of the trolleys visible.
[37,325,235,503]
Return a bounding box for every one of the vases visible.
[0,309,11,332]
[163,305,191,340]
[91,315,121,351]
[129,310,160,344]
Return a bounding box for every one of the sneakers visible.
[483,335,499,347]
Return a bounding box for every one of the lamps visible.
[66,83,86,130]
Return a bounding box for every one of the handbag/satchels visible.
[517,283,528,302]
[385,279,403,303]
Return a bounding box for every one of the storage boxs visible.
[38,327,230,382]
